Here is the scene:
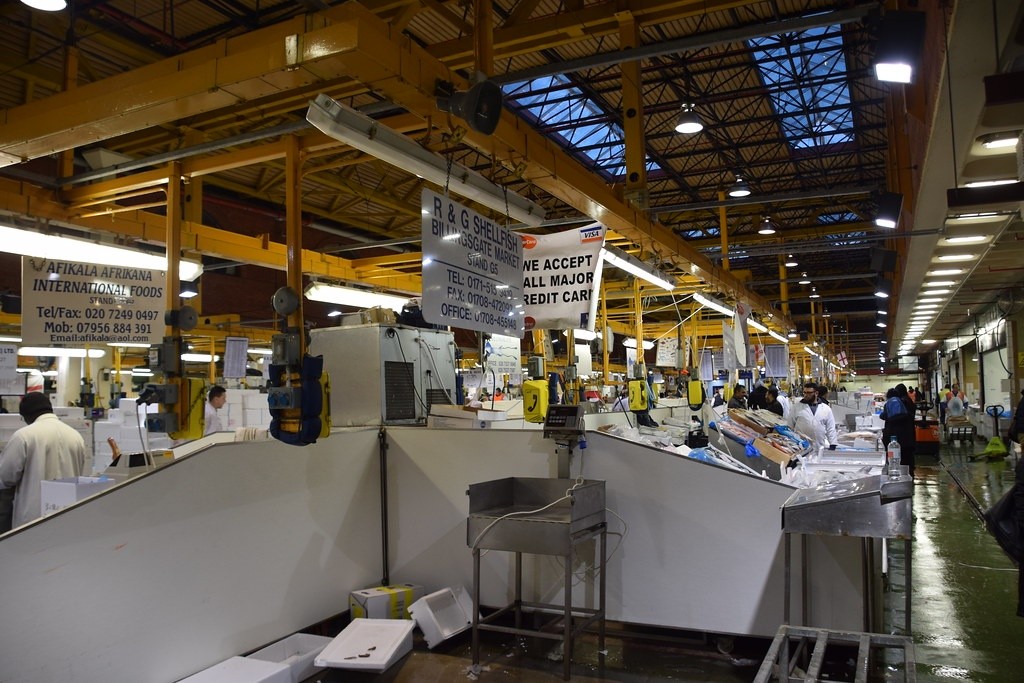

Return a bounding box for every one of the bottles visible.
[887,436,901,481]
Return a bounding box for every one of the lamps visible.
[307,93,547,228]
[0,221,204,283]
[728,162,751,197]
[758,212,776,234]
[975,129,1024,148]
[694,289,856,377]
[563,328,655,350]
[179,281,199,297]
[304,282,410,314]
[876,193,905,229]
[785,255,846,332]
[602,243,679,291]
[873,10,927,85]
[871,249,897,374]
[675,76,704,133]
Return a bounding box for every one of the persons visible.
[613,393,630,411]
[728,379,829,417]
[203,385,227,435]
[0,392,86,528]
[479,388,489,401]
[711,388,723,407]
[787,382,838,450]
[879,383,968,480]
[494,388,505,399]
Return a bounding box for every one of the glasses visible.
[803,391,815,394]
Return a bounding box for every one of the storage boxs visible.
[175,583,483,683]
[975,411,1013,441]
[431,404,506,421]
[0,389,273,517]
[639,387,885,467]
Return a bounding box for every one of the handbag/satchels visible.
[984,478,1023,564]
[885,397,908,420]
